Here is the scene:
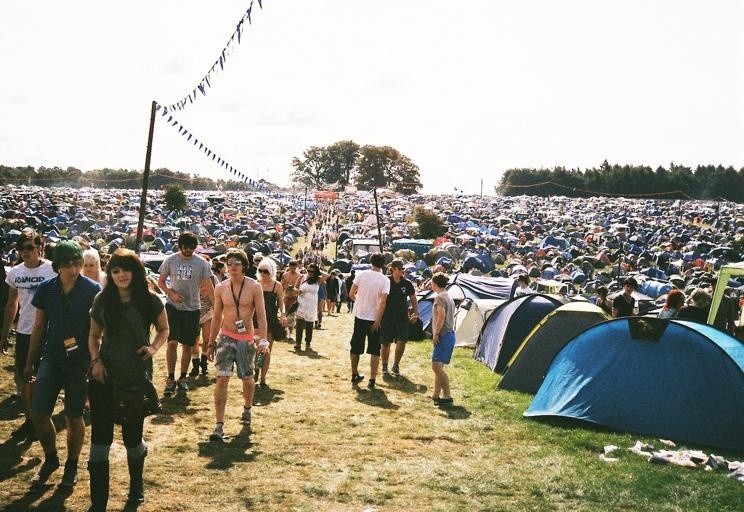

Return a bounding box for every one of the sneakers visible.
[210,425,224,439]
[391,367,399,375]
[63,466,78,485]
[368,378,375,386]
[382,367,387,373]
[178,379,189,390]
[429,395,453,404]
[164,381,176,392]
[352,375,364,382]
[31,459,59,485]
[241,412,250,424]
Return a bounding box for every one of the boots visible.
[190,358,199,376]
[200,354,208,373]
[86,461,109,512]
[127,447,146,501]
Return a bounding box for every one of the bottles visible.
[255,352,265,370]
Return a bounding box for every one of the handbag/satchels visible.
[112,383,165,424]
[289,301,299,314]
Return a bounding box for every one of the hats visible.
[392,260,405,270]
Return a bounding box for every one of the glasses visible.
[63,256,83,268]
[259,269,269,273]
[16,244,39,252]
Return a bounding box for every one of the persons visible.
[86,247,170,511]
[22,239,102,487]
